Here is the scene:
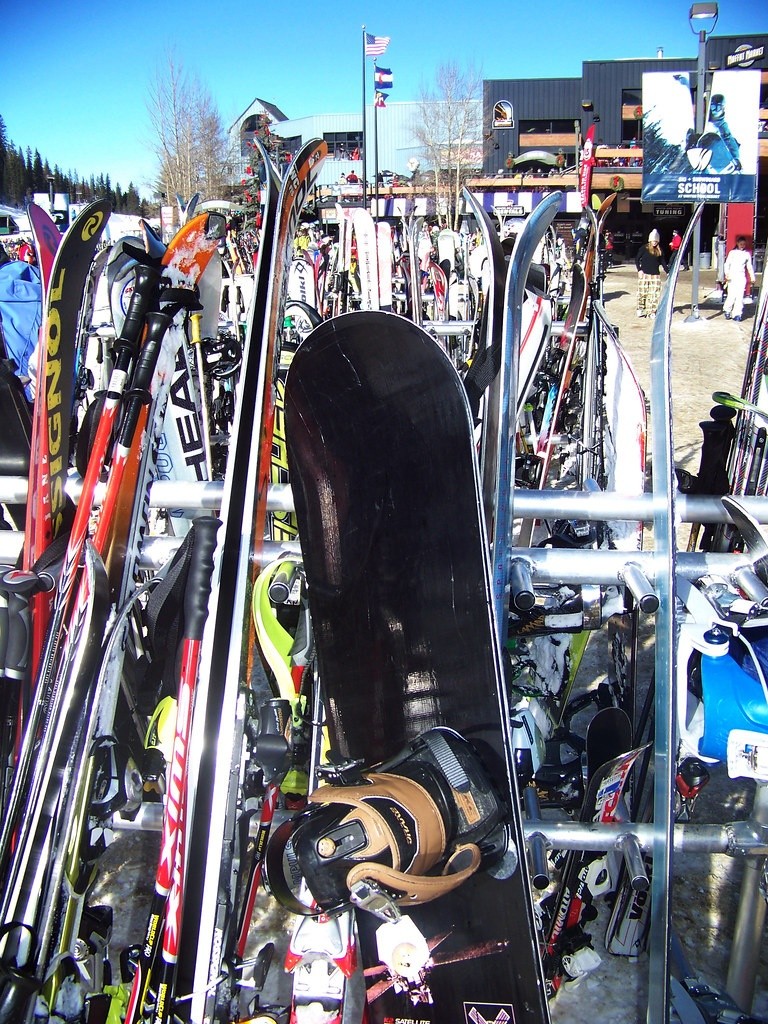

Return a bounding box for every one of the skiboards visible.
[0,136,768,1024]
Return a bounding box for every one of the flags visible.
[375,90,389,107]
[365,32,390,56]
[375,66,393,88]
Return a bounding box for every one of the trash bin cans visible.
[50,210,69,232]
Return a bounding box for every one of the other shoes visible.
[683,266,690,272]
[638,310,656,319]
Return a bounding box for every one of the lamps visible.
[483,129,490,135]
[581,99,592,107]
[708,61,721,71]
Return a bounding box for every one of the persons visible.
[724,236,755,321]
[763,125,768,132]
[353,146,359,160]
[475,167,558,179]
[340,170,358,202]
[392,175,400,187]
[601,144,642,167]
[338,142,346,161]
[672,74,741,170]
[667,229,690,271]
[378,172,385,188]
[635,229,669,319]
[604,230,614,269]
[0,237,37,264]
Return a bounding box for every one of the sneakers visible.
[724,312,742,322]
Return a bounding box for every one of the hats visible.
[648,229,660,243]
